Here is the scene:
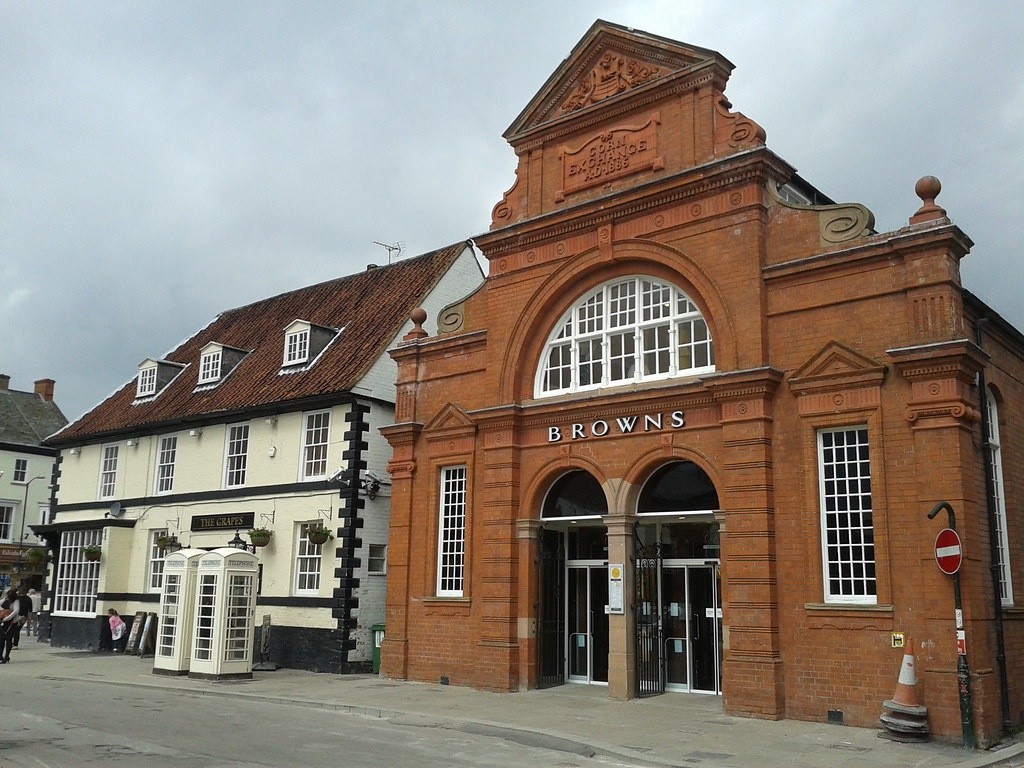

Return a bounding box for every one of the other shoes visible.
[11,646,18,650]
[113,649,117,651]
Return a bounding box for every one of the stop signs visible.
[935,528,963,576]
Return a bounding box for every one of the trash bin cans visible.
[368,624,385,674]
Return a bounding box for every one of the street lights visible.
[17,476,47,576]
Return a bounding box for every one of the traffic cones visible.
[879,635,932,744]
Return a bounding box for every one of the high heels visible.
[0,657,3,661]
[0,657,10,664]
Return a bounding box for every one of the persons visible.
[0,587,40,664]
[108,608,126,652]
